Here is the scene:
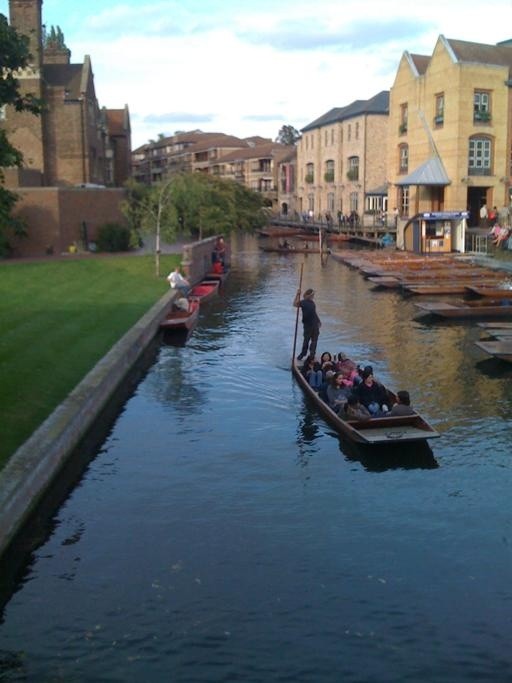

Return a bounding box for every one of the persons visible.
[308,209,315,224]
[392,207,400,228]
[166,265,190,298]
[173,290,190,313]
[301,351,414,424]
[304,240,308,249]
[283,239,295,249]
[377,210,386,229]
[292,288,322,361]
[215,236,227,273]
[479,204,512,247]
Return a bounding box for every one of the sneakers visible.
[297,352,306,360]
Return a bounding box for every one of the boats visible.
[159,261,233,330]
[292,353,441,446]
[257,225,349,255]
[327,247,512,362]
